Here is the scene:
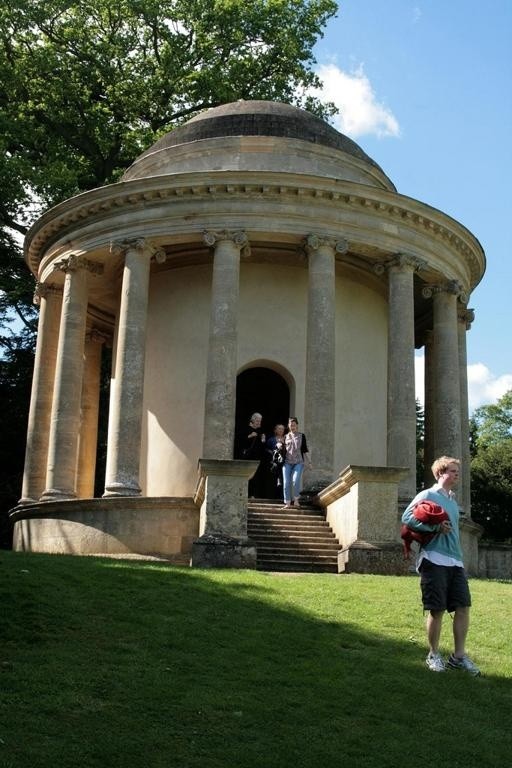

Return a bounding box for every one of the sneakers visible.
[426,651,482,676]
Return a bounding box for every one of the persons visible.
[400,456,481,677]
[243,413,313,510]
